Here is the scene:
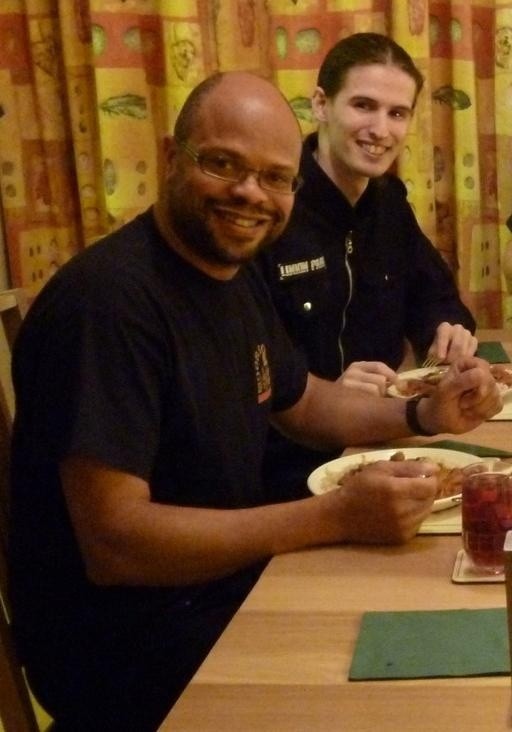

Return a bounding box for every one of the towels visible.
[416,341,512,368]
[347,606,512,681]
[418,440,512,458]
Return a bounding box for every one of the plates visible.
[306,448,481,513]
[386,366,512,399]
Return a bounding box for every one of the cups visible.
[461,460,511,575]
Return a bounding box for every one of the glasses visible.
[171,134,305,195]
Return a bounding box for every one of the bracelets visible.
[406,390,436,437]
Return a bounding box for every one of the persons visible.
[2,72,504,729]
[251,31,480,399]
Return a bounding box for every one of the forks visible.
[421,356,441,368]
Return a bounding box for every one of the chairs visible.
[0,290,71,732]
